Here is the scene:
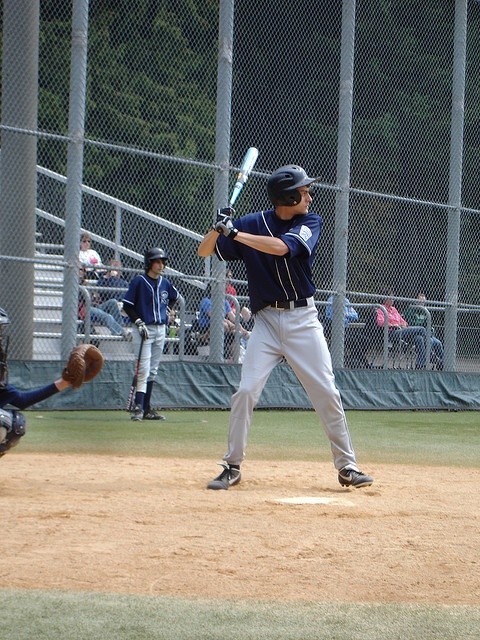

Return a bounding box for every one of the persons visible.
[375,290,443,371]
[200,281,253,365]
[98,261,133,326]
[78,268,131,341]
[401,293,443,358]
[239,305,254,331]
[226,269,238,314]
[324,296,383,369]
[167,310,199,343]
[0,306,73,458]
[123,247,178,421]
[196,165,374,491]
[79,234,107,273]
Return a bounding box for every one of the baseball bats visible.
[215,146,263,232]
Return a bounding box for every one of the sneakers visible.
[144,411,166,420]
[207,463,241,489]
[131,404,144,421]
[120,327,132,337]
[338,464,373,488]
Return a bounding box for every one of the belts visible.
[271,299,307,308]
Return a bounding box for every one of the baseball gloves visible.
[63,344,103,386]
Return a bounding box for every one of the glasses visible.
[83,241,90,242]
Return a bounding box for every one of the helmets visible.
[144,247,168,268]
[267,165,322,206]
[0,307,12,363]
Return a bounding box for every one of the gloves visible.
[165,306,171,325]
[212,207,237,230]
[216,216,238,240]
[135,318,149,341]
